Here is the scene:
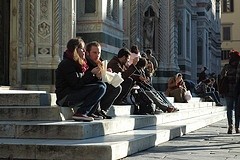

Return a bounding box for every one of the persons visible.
[83,41,121,120]
[196,67,223,106]
[168,73,187,103]
[221,50,240,133]
[219,63,229,97]
[107,45,179,115]
[55,38,106,122]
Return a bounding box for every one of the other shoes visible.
[236,129,240,133]
[88,113,103,119]
[72,112,93,121]
[228,125,232,134]
[95,110,112,119]
[216,102,223,106]
[168,107,174,112]
